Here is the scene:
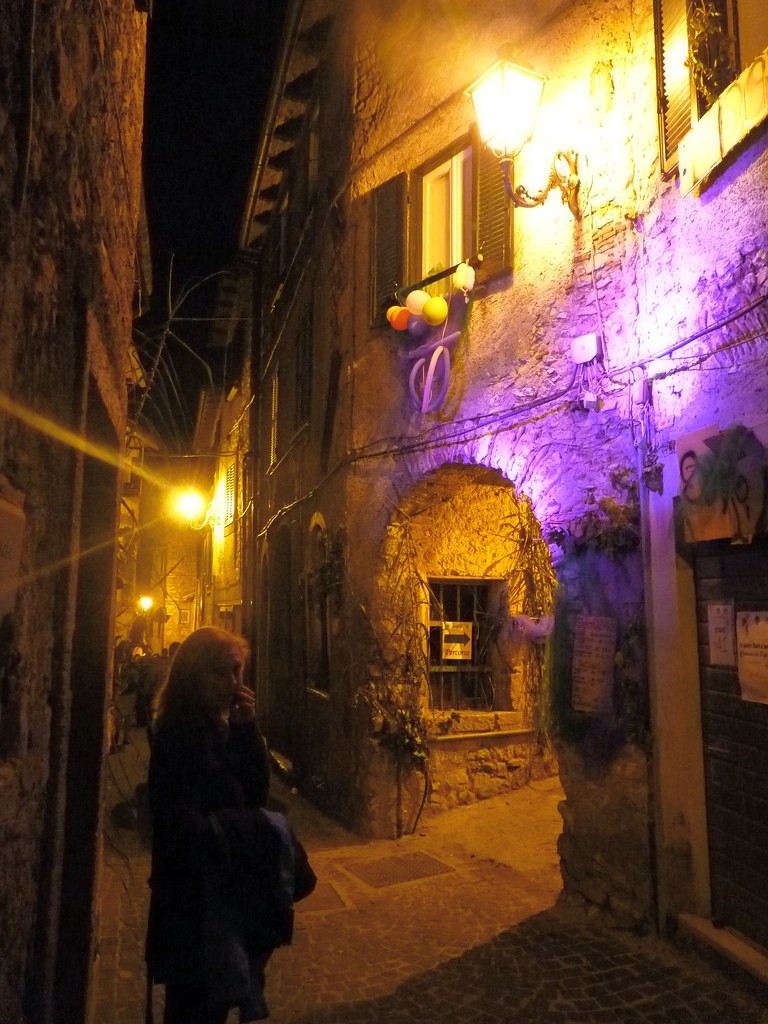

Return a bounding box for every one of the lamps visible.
[463,41,581,219]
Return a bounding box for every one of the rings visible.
[248,697,255,703]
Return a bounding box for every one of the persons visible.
[111,640,182,750]
[142,626,299,1024]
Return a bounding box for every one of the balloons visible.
[386,288,449,338]
